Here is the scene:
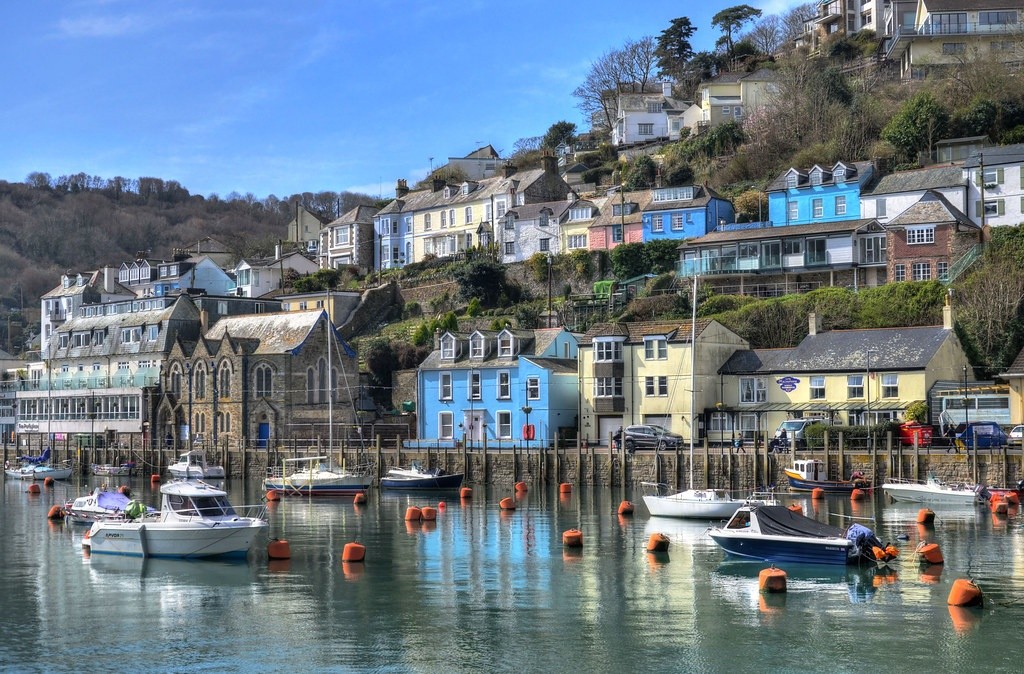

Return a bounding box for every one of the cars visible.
[1007,424,1024,448]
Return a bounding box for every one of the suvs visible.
[622,424,684,452]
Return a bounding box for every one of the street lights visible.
[963,365,970,457]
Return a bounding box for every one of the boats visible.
[78,452,272,565]
[707,491,899,569]
[879,471,1021,516]
[379,458,466,492]
[55,483,161,524]
[90,462,136,477]
[165,450,226,480]
[783,457,880,494]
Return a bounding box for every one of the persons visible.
[778,428,787,453]
[943,424,958,454]
[166,432,173,450]
[735,433,745,453]
[615,426,623,446]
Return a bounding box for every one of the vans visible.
[773,417,827,450]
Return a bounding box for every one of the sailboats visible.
[5,340,73,482]
[641,273,801,521]
[262,288,376,500]
